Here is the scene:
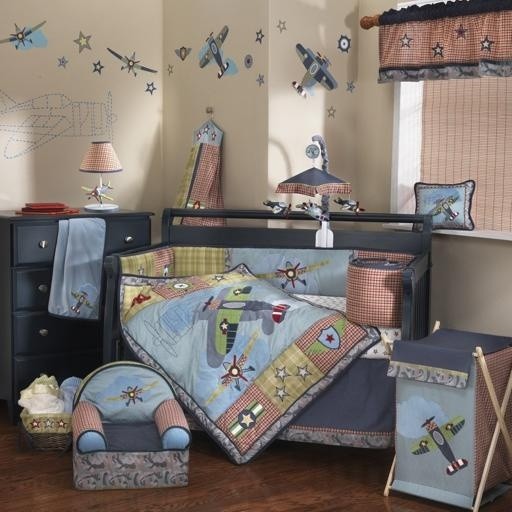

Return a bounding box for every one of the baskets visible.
[20,408,72,450]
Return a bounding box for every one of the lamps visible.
[79,139,124,213]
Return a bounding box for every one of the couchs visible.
[101,207,434,456]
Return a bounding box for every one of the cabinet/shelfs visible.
[1,208,156,426]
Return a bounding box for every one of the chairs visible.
[69,362,189,490]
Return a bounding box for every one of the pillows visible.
[411,179,475,232]
